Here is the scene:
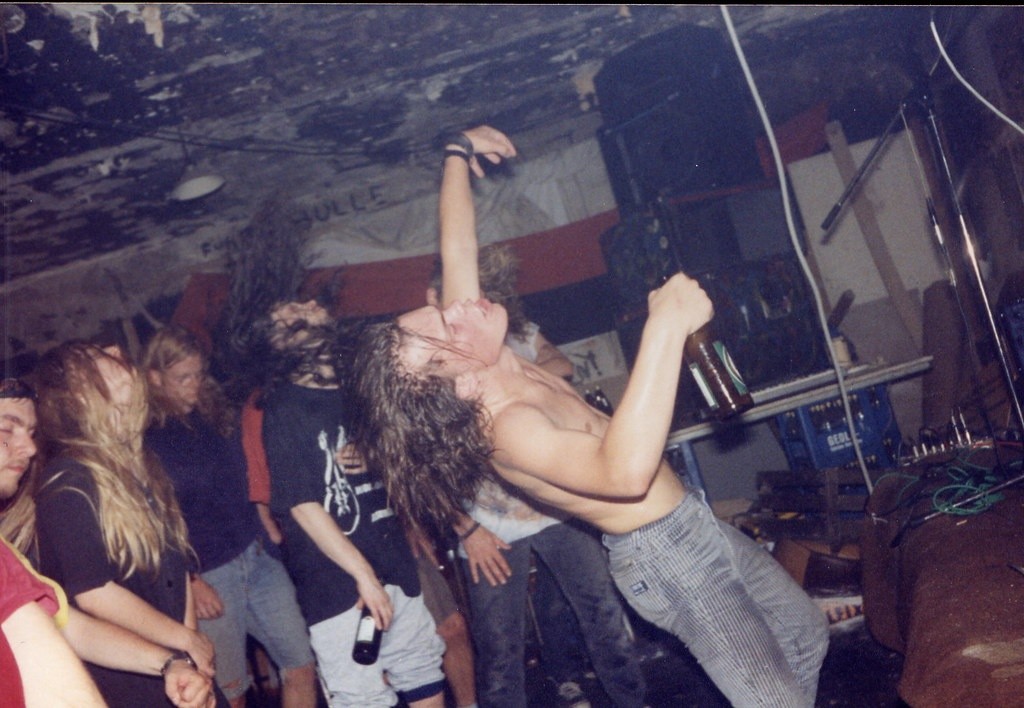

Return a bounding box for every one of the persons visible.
[1,238,674,708]
[347,120,831,708]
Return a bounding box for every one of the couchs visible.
[861,440,1024,708]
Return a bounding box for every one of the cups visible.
[825,336,853,371]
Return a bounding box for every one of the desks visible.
[663,353,933,506]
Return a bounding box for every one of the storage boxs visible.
[776,384,906,519]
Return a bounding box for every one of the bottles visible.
[776,386,897,468]
[656,271,752,422]
[585,384,614,415]
[352,580,386,666]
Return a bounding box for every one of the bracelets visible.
[458,520,481,543]
[443,133,476,160]
[160,655,194,677]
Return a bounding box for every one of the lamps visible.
[169,124,224,203]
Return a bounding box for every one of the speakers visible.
[598,25,833,420]
